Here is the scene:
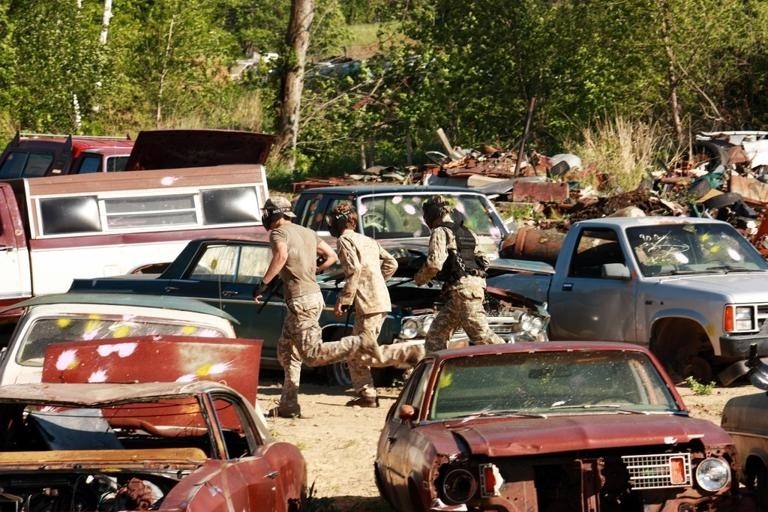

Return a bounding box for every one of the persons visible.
[313,201,428,408]
[250,196,387,419]
[412,193,509,361]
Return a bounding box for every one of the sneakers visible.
[270,405,301,417]
[412,344,425,368]
[360,330,384,363]
[347,397,377,407]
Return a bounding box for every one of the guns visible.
[258,255,326,314]
[387,250,458,289]
[344,301,356,334]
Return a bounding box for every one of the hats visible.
[263,196,297,218]
[427,195,453,213]
